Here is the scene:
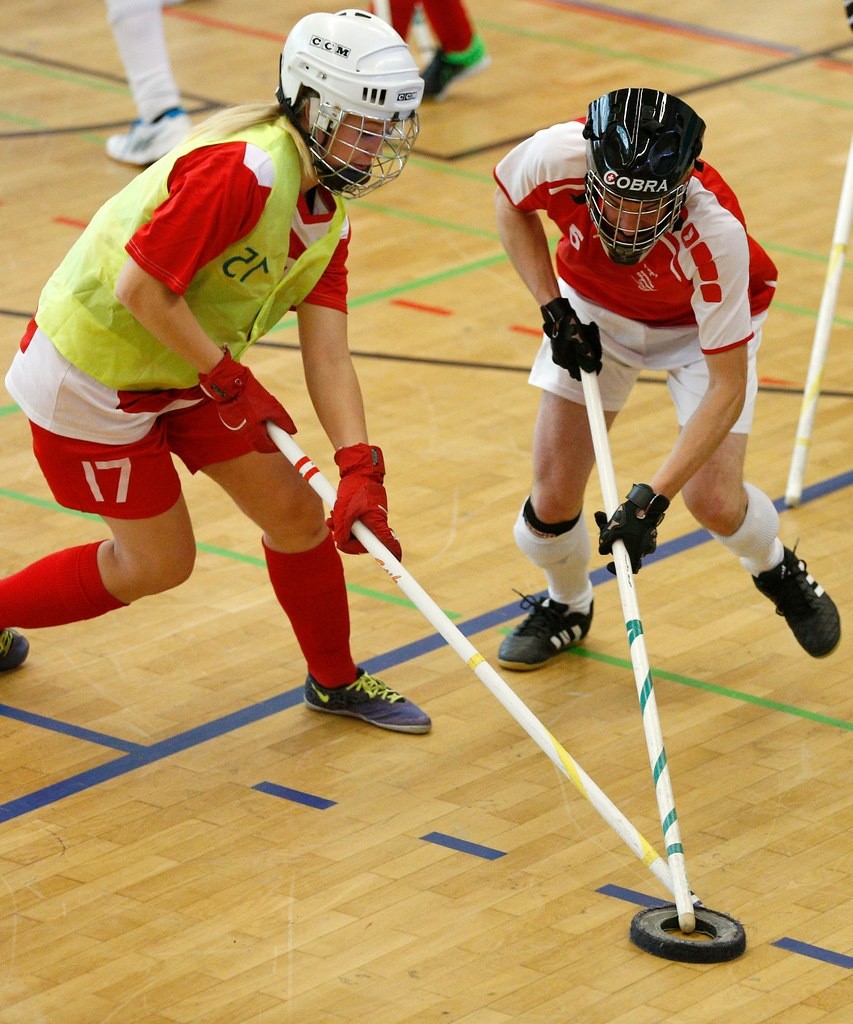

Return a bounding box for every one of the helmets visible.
[278,6,425,201]
[585,88,706,263]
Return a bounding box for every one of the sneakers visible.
[305,669,432,737]
[104,110,192,165]
[0,626,30,671]
[752,546,841,659]
[420,34,490,102]
[499,597,593,672]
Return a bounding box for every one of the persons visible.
[484,86,843,670]
[99,0,199,167]
[371,0,489,102]
[0,5,443,735]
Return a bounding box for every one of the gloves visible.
[594,483,669,575]
[540,295,604,380]
[328,444,402,565]
[197,345,297,454]
